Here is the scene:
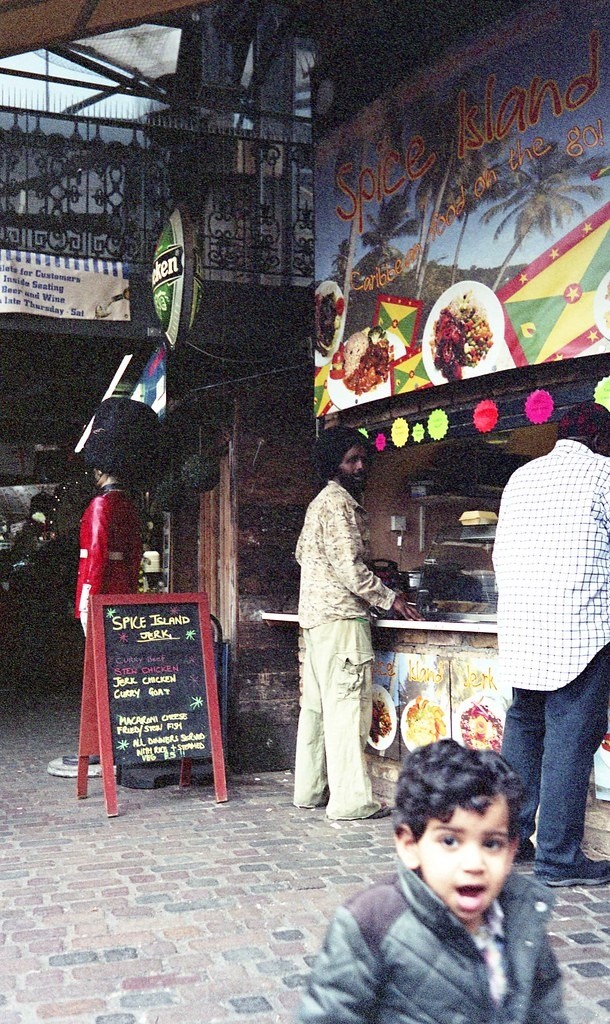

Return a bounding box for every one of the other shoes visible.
[533,857,610,886]
[513,847,538,866]
[368,802,390,820]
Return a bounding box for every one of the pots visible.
[393,560,465,593]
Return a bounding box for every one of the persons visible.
[492,403,610,887]
[290,428,426,823]
[298,738,569,1023]
[46,397,169,778]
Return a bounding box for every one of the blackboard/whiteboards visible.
[89,591,225,765]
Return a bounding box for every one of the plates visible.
[454,696,507,748]
[422,280,504,386]
[315,281,345,369]
[599,732,610,767]
[399,698,449,750]
[328,330,406,409]
[593,270,610,340]
[367,684,396,752]
